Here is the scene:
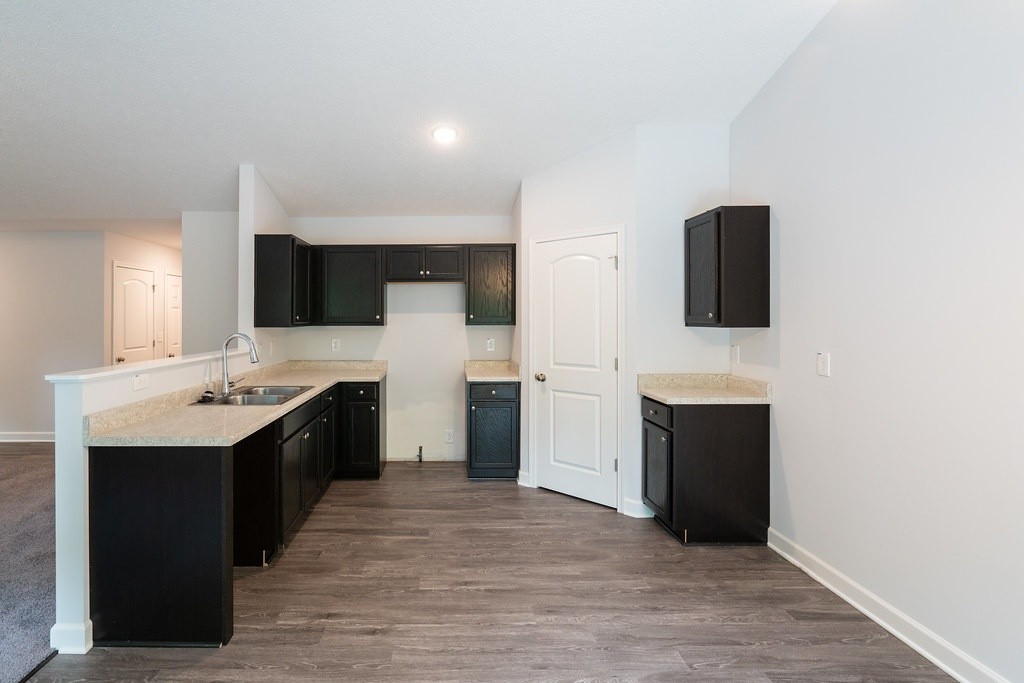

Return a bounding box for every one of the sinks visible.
[215,395,286,412]
[239,387,303,395]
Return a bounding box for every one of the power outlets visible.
[444,430,454,442]
[133,373,145,390]
[331,338,341,351]
[731,344,739,363]
[486,336,495,350]
[817,351,830,376]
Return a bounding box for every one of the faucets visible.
[221,333,259,395]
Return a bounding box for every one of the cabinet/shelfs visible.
[466,243,516,324]
[387,243,465,282]
[641,396,771,546]
[465,376,520,480]
[683,204,771,328]
[254,234,310,326]
[233,382,338,566]
[310,243,387,326]
[339,374,387,480]
